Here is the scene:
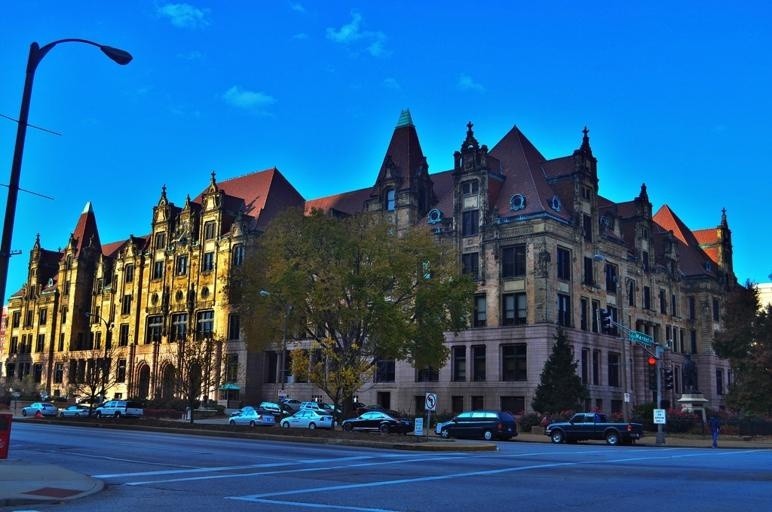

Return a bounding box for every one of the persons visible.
[709,415,721,448]
[541,415,549,435]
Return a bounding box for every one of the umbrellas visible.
[217,383,240,399]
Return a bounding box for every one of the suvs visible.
[299,400,334,416]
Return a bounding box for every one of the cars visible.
[343,408,415,434]
[434,409,519,440]
[60,405,96,418]
[231,405,264,418]
[260,401,283,418]
[20,402,59,418]
[279,406,334,431]
[12,391,22,398]
[38,390,48,400]
[354,401,366,408]
[359,403,384,410]
[281,398,301,412]
[320,401,334,411]
[228,409,276,428]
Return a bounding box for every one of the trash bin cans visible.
[0,412,13,459]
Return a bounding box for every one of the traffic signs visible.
[631,330,653,346]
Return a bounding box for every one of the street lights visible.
[0,36,132,364]
[86,311,130,402]
[260,288,290,396]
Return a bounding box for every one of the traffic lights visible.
[648,356,657,388]
[603,312,612,333]
[667,371,673,389]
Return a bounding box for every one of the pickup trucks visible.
[544,411,647,445]
[96,399,145,419]
